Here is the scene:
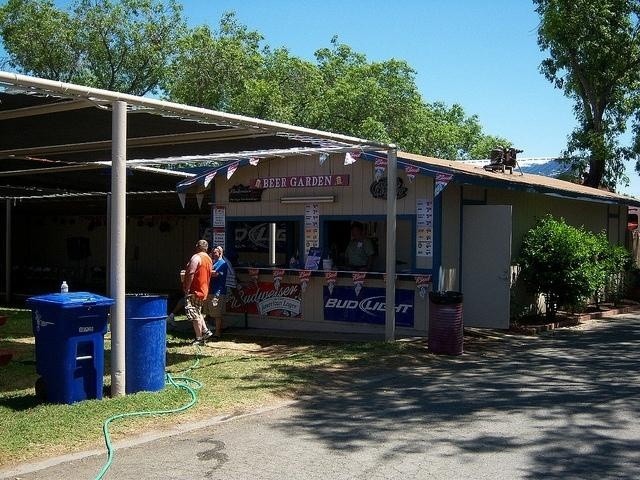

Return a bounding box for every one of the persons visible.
[212,245,227,340]
[181,239,213,346]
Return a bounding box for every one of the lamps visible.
[280,195,334,204]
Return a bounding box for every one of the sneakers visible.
[186,328,220,346]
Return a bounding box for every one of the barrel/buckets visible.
[126,293,169,394]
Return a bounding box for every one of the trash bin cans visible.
[431,291,462,354]
[27,292,114,401]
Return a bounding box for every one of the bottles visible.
[60,280,69,305]
